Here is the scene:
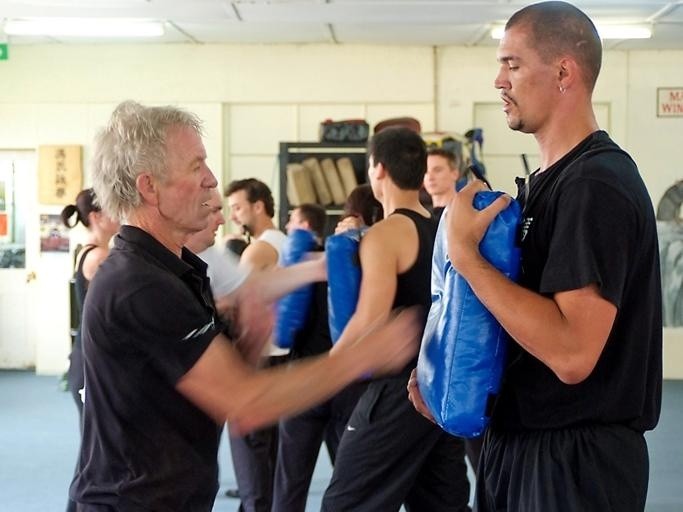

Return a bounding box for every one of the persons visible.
[65,101,424,511]
[62,188,120,438]
[182,125,472,511]
[407,1,662,512]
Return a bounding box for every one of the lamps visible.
[2,16,164,37]
[489,20,655,40]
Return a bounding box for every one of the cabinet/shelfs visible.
[279,143,370,239]
